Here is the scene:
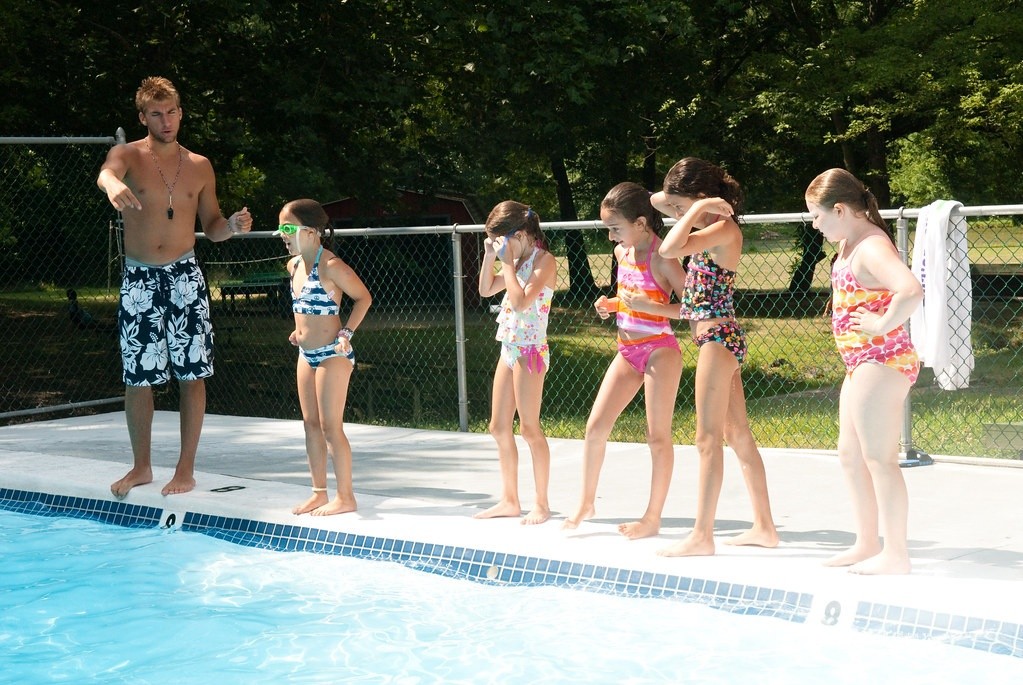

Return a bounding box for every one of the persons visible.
[801,167,924,578]
[273,198,374,517]
[560,180,688,541]
[474,201,560,527]
[649,155,781,557]
[97,76,253,499]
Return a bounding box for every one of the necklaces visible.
[143,136,182,220]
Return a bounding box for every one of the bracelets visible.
[337,326,354,340]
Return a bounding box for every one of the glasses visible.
[278,225,309,234]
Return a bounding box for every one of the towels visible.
[908,198,976,393]
